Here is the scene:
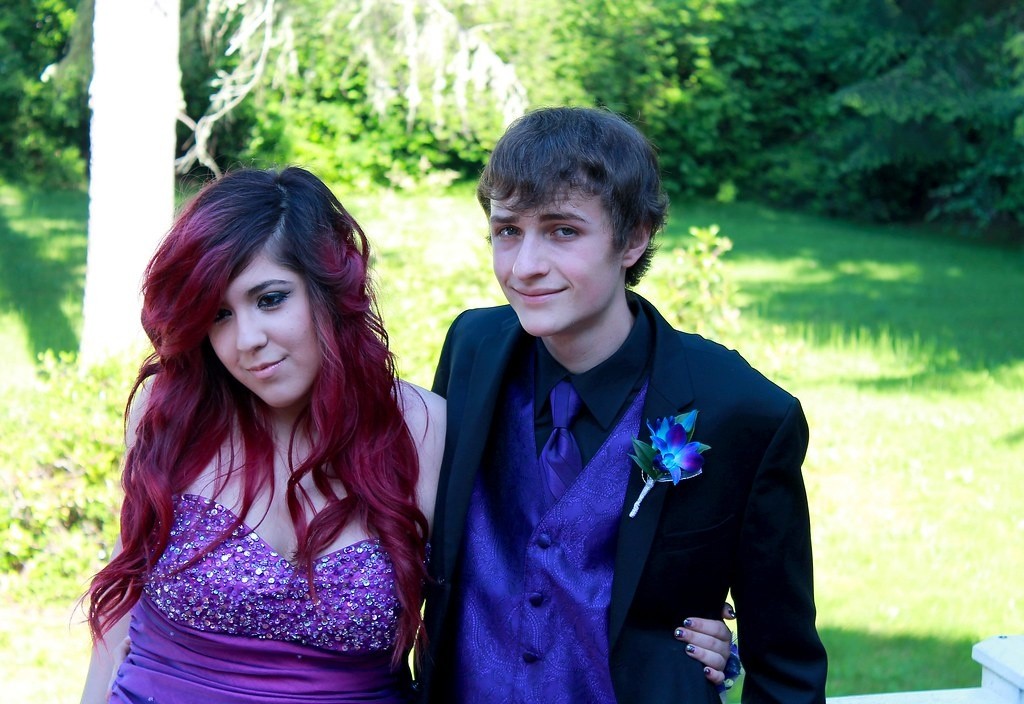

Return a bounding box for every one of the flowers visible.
[626,409,711,520]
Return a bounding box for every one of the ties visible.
[538,379,583,512]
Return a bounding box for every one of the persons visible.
[412,105,831,704]
[78,164,742,703]
